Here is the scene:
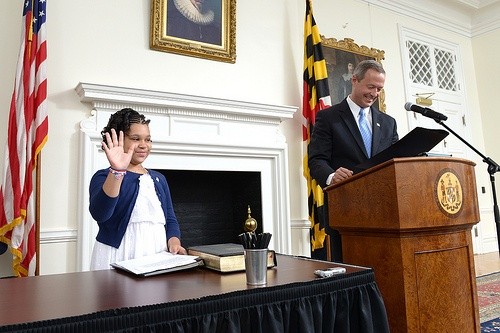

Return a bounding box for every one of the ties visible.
[359,108,372,159]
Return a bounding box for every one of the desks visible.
[0,252,392,333]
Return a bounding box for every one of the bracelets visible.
[108,166,127,179]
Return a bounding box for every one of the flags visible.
[303,0,333,265]
[0,0,49,277]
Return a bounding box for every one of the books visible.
[188,243,277,273]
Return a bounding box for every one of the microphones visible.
[405,103,448,121]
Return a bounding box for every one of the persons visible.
[89,108,187,272]
[310,59,400,265]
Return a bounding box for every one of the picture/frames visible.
[320,32,388,114]
[149,0,238,64]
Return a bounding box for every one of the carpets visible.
[476,271,500,333]
